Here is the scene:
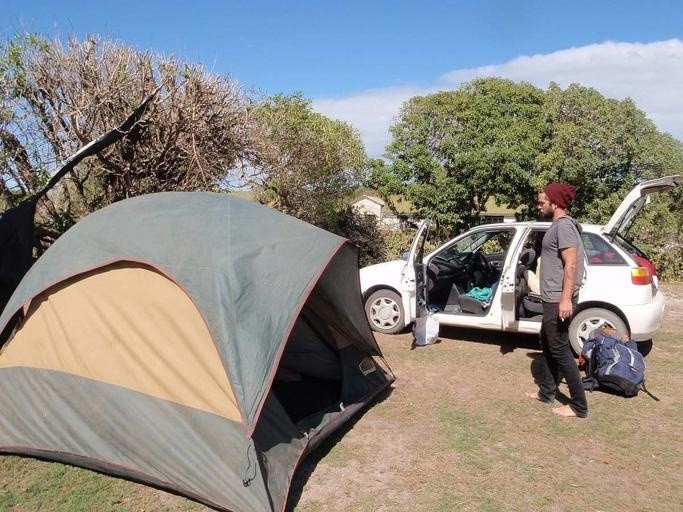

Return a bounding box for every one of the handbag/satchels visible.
[413,317,440,347]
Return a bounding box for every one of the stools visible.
[520,296,543,315]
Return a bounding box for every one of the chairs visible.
[457,249,535,314]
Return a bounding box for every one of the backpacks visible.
[580,323,646,398]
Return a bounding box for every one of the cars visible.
[358,174,678,360]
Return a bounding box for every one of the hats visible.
[543,182,577,210]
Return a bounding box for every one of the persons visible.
[528,183,587,417]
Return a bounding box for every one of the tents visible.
[0,191,396,512]
